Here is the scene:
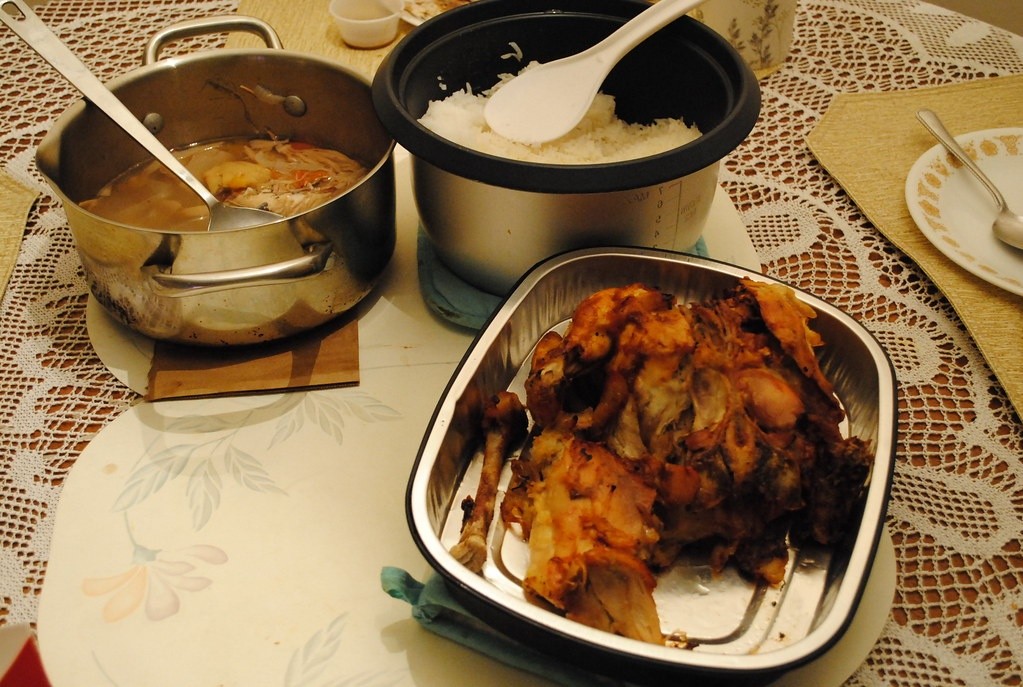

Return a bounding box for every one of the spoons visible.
[915,108,1023,250]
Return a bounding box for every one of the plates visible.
[905,127,1023,298]
[382,0,478,27]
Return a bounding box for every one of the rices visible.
[416,40,704,165]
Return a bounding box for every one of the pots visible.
[372,0,761,297]
[37,14,398,348]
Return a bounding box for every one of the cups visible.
[686,0,796,81]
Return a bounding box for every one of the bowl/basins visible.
[405,245,900,687]
[328,0,405,50]
